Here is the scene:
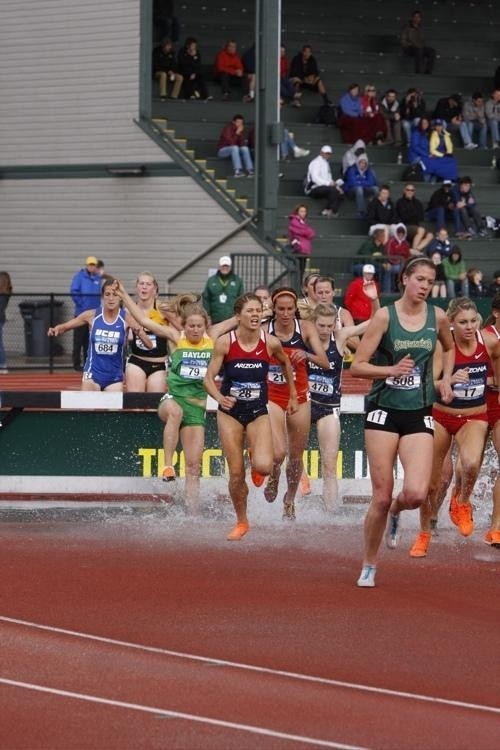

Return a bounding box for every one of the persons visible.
[155,38,500,301]
[48,256,500,587]
[153,0,181,48]
[400,10,435,76]
[0,269,13,375]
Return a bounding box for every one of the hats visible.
[434,119,444,126]
[363,264,375,274]
[321,145,333,153]
[87,257,97,265]
[220,256,233,268]
[442,179,453,187]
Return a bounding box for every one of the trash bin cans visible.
[19,299,64,356]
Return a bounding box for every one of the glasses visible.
[405,188,415,192]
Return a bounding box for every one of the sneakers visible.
[321,208,339,218]
[247,170,256,178]
[492,141,499,150]
[463,142,478,150]
[359,210,366,218]
[377,139,385,147]
[227,446,499,588]
[162,466,175,482]
[244,95,255,102]
[293,147,310,159]
[234,171,247,178]
[292,99,302,107]
[222,95,232,102]
[438,221,499,239]
